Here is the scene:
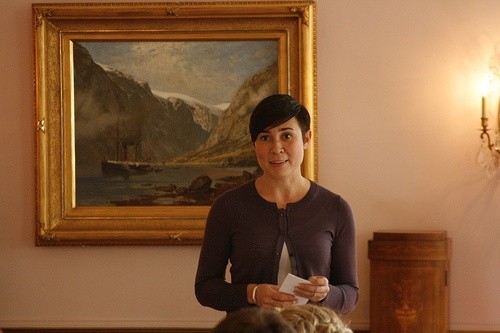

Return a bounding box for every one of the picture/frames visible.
[30,0,320,246]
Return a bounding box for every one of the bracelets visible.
[253,284,261,305]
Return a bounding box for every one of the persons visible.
[192,93,360,316]
[210,308,295,333]
[276,305,355,333]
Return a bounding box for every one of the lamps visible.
[473,69,500,160]
